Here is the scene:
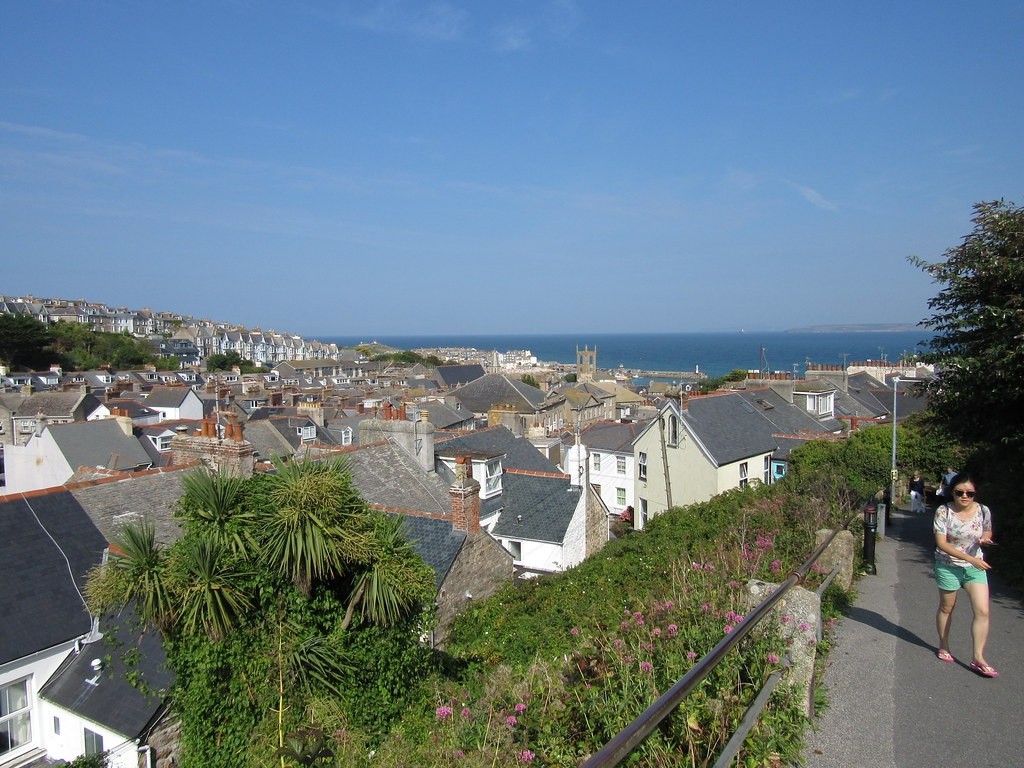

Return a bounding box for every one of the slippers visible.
[935,649,954,662]
[970,662,999,677]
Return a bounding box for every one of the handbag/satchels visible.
[936,486,945,496]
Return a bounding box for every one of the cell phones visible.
[982,541,1000,547]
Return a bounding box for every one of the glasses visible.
[953,490,976,498]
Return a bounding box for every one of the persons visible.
[940,467,957,498]
[910,470,926,513]
[934,473,999,678]
